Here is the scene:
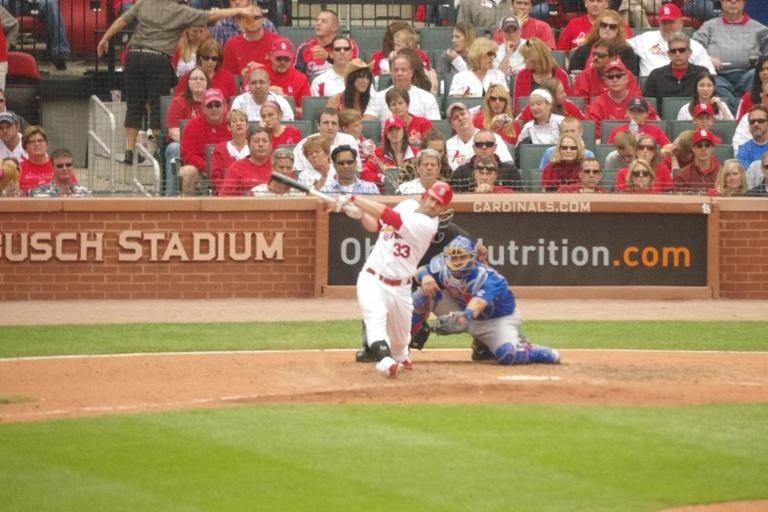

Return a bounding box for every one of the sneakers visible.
[354,347,377,363]
[375,355,398,377]
[532,346,560,365]
[401,357,413,371]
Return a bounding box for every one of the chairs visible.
[6,16,43,126]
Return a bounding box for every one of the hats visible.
[626,97,650,113]
[342,56,376,84]
[200,89,225,106]
[654,3,683,21]
[689,128,719,146]
[691,104,716,116]
[269,37,296,60]
[501,16,519,32]
[445,103,466,117]
[0,112,18,125]
[384,115,405,134]
[604,61,625,73]
[529,88,554,104]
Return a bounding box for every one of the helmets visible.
[447,236,479,274]
[423,179,454,209]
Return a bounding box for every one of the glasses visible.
[606,73,627,80]
[670,47,688,55]
[335,159,355,166]
[206,103,223,110]
[582,169,600,175]
[201,54,219,63]
[474,164,497,172]
[474,141,495,148]
[748,118,767,125]
[333,46,351,53]
[637,144,654,151]
[489,96,505,102]
[55,161,74,169]
[559,145,578,151]
[485,51,498,59]
[599,22,618,31]
[630,170,649,177]
[591,50,611,58]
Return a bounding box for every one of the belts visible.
[364,265,413,287]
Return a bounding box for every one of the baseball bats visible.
[271,171,337,202]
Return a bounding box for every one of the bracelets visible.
[417,271,429,282]
[350,195,354,202]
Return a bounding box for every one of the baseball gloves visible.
[430,312,470,334]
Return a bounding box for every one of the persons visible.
[409,234,562,361]
[354,207,497,364]
[0,1,767,198]
[323,179,455,376]
